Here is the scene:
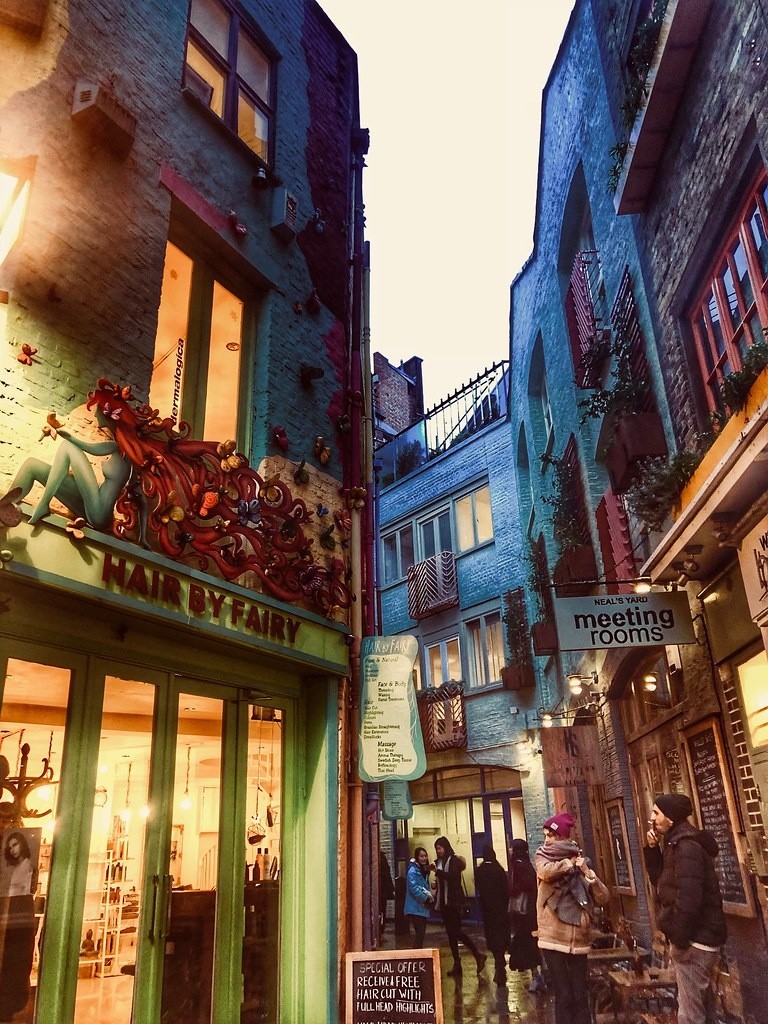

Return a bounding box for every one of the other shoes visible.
[529,981,540,991]
[477,953,487,973]
[447,959,462,975]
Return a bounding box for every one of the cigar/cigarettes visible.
[647,821,653,824]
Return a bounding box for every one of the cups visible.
[648,967,659,981]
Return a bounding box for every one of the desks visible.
[606,966,677,1024]
[587,943,650,1024]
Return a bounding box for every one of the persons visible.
[643,794,726,1024]
[431,837,487,975]
[9,403,132,526]
[404,847,431,949]
[508,839,547,992]
[535,813,609,1024]
[379,851,396,942]
[476,844,510,985]
[82,930,98,978]
[3,832,32,896]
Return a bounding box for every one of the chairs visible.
[650,929,666,970]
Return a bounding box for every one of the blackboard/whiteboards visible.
[342,948,446,1024]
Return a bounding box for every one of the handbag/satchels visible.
[506,891,527,915]
[459,901,472,916]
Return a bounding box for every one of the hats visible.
[544,812,575,839]
[655,794,694,824]
[483,844,494,858]
[514,838,529,852]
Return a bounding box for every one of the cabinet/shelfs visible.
[76,848,112,980]
[92,832,128,978]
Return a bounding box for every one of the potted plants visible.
[576,330,667,495]
[524,537,556,654]
[539,454,597,593]
[497,603,535,689]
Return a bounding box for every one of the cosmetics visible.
[245,847,271,880]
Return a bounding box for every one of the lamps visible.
[710,510,732,543]
[564,670,598,695]
[672,561,690,588]
[682,544,704,572]
[539,711,557,729]
